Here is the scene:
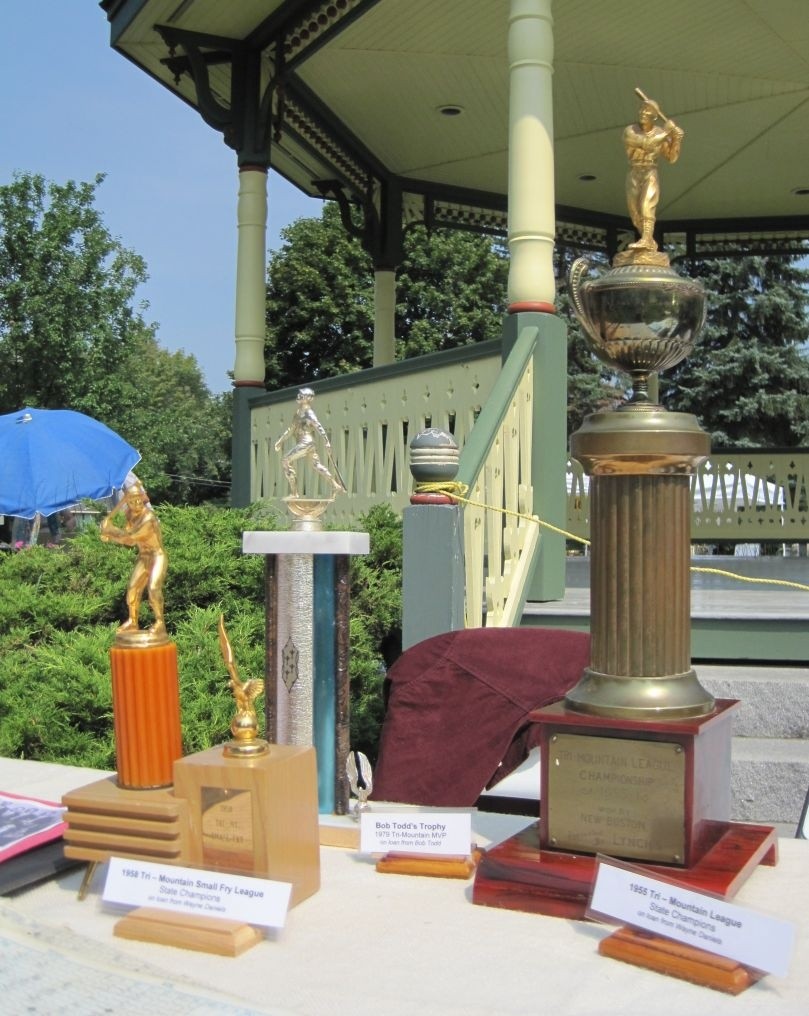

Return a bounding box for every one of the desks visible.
[0,756,809,1016]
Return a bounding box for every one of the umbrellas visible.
[0,404,143,521]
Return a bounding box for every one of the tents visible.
[566,454,788,561]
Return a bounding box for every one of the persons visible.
[275,388,346,499]
[100,480,166,641]
[622,99,686,252]
